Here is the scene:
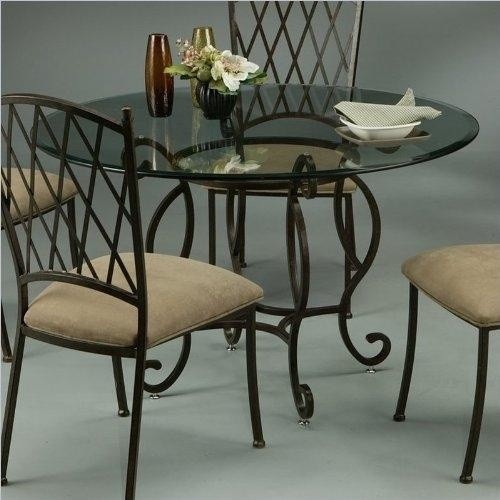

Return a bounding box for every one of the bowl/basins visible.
[340,113,422,138]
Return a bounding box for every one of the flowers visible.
[170,151,263,175]
[163,38,269,96]
[194,82,237,119]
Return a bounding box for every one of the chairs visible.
[1,166,80,361]
[0,93,265,500]
[393,243,499,482]
[208,1,363,319]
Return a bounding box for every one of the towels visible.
[396,86,415,106]
[335,100,442,128]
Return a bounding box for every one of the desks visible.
[29,84,479,428]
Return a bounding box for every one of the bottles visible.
[151,119,172,174]
[144,33,175,118]
[191,26,217,107]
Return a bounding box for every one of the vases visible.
[196,122,238,159]
